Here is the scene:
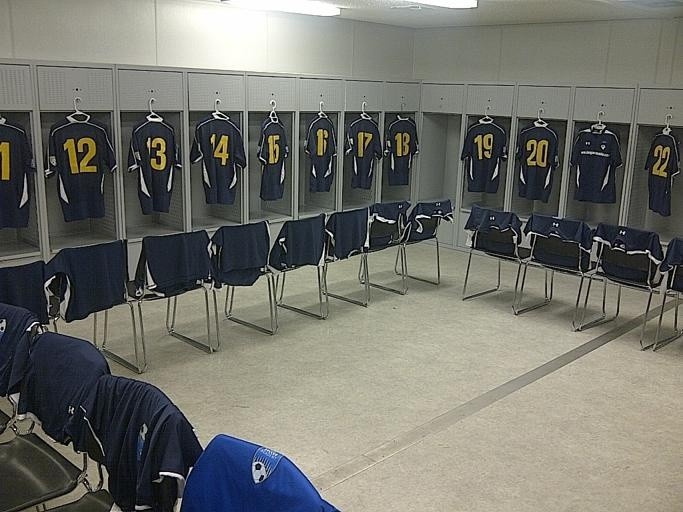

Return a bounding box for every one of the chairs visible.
[517,212,592,331]
[329,200,412,305]
[47,237,140,377]
[284,209,368,318]
[0,263,45,345]
[365,196,451,292]
[100,230,215,374]
[3,302,349,512]
[174,221,273,356]
[230,212,328,339]
[578,222,664,350]
[652,237,683,350]
[460,204,522,315]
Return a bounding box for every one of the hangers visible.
[662,114,674,133]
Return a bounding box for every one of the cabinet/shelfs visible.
[188,75,243,234]
[297,80,346,225]
[342,83,385,212]
[248,77,298,232]
[378,84,419,209]
[623,92,683,240]
[505,86,570,225]
[460,86,515,213]
[1,61,46,266]
[118,69,186,247]
[416,86,464,206]
[35,67,123,259]
[564,88,638,230]
